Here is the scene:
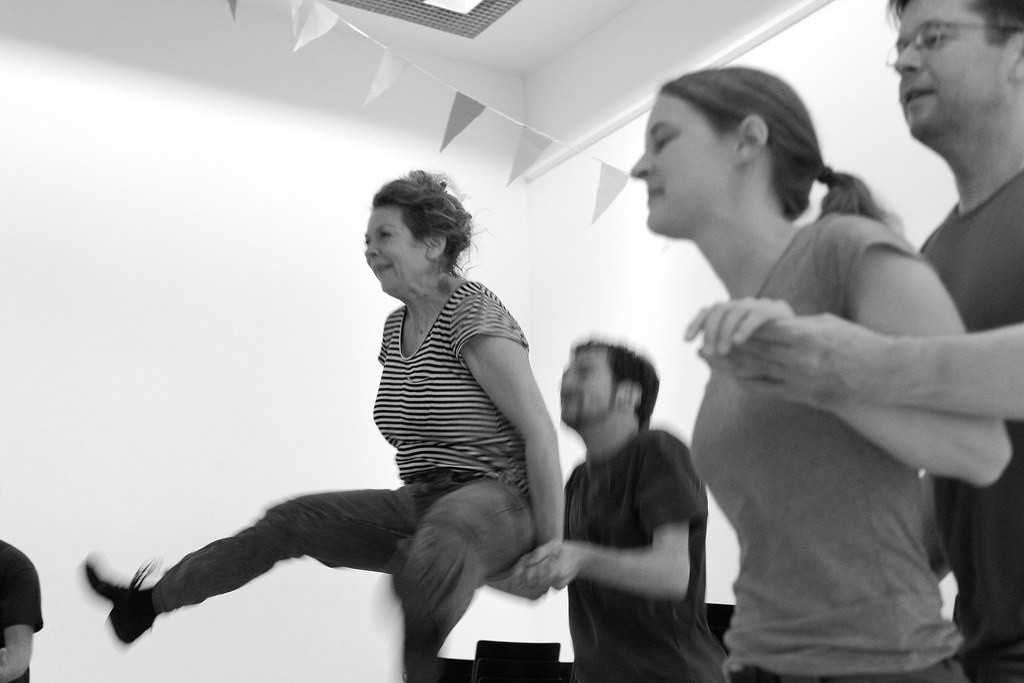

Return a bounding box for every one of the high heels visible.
[86,558,158,643]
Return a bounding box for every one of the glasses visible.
[885,20,1023,67]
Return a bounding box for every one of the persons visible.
[698,1,1024,683]
[631,67,1011,681]
[486,342,727,683]
[84,170,562,683]
[0,541,44,683]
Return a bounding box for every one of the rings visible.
[518,583,524,590]
